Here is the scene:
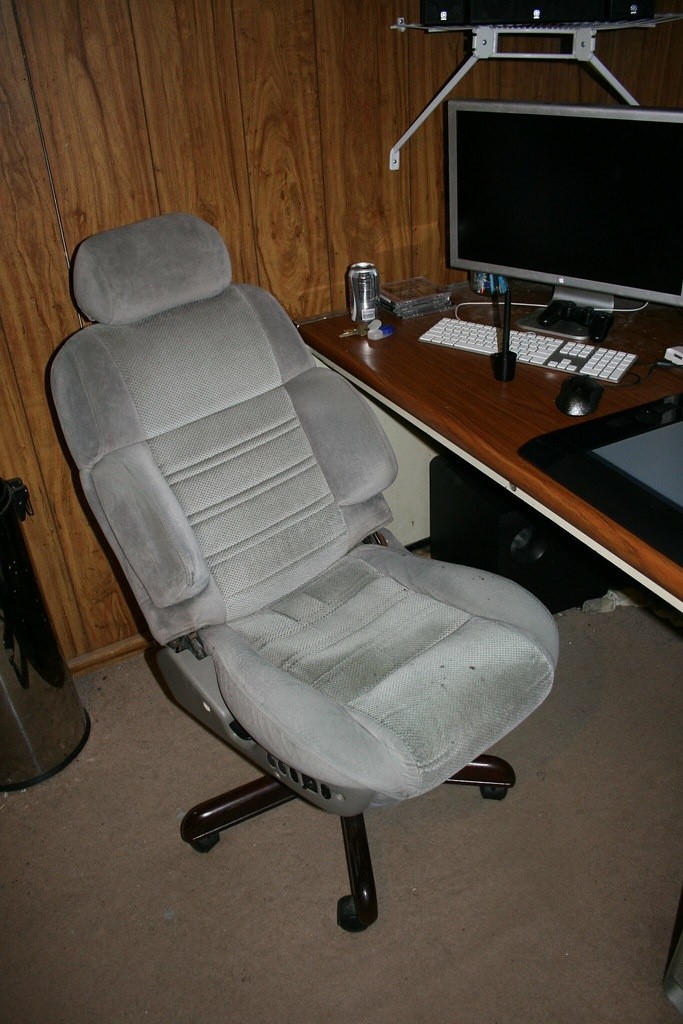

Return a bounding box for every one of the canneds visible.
[348,262,379,323]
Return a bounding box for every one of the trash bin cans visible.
[0,478,92,793]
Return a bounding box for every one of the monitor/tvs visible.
[448,100,683,340]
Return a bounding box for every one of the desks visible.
[296,285,683,613]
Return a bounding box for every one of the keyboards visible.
[418,318,638,384]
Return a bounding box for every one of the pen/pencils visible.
[502,287,513,353]
[471,270,501,296]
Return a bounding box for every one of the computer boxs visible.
[429,451,636,614]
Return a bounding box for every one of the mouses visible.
[556,374,604,416]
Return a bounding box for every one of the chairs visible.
[49,211,560,933]
